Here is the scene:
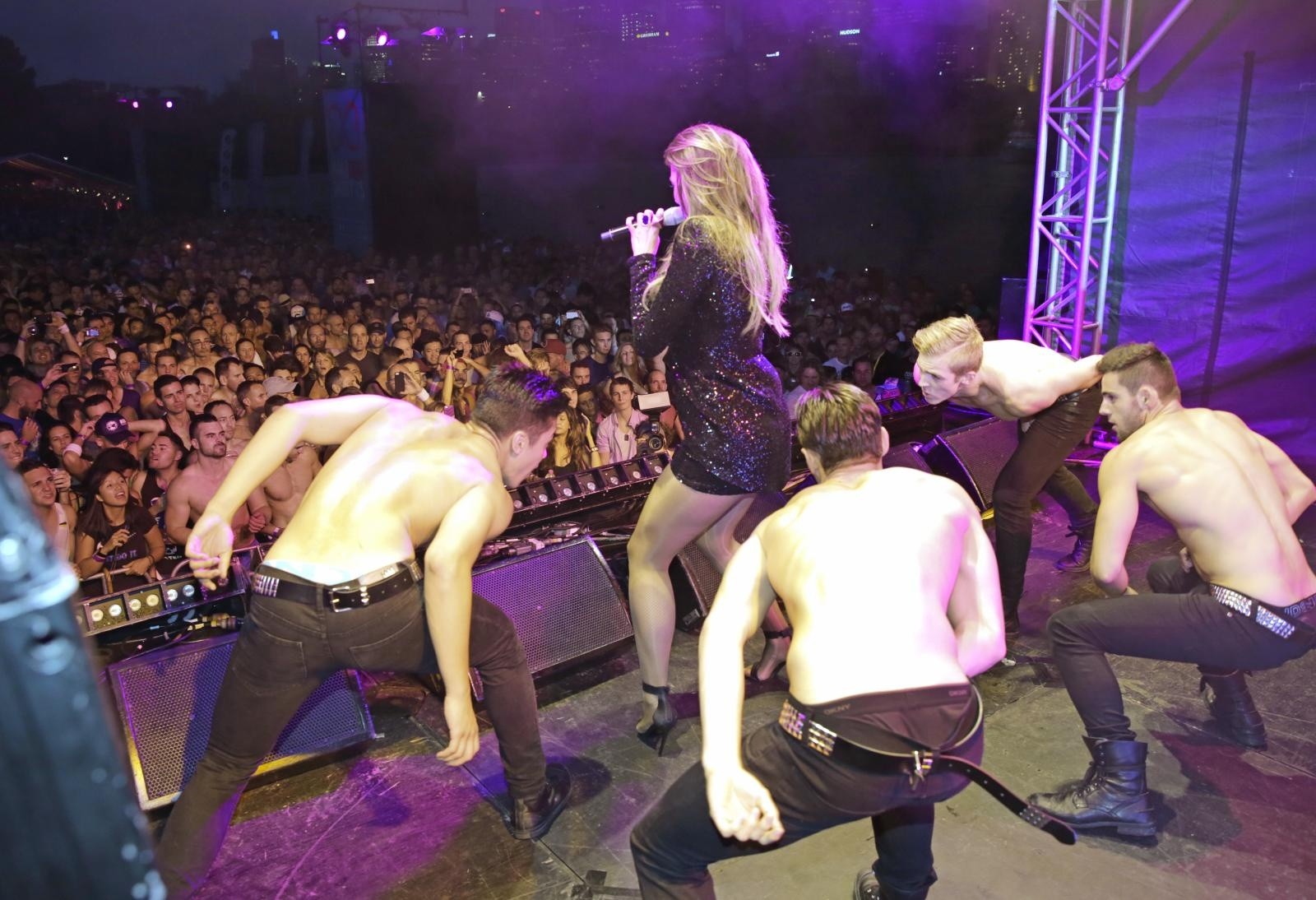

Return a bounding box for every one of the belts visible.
[773,693,1080,848]
[1209,583,1316,651]
[1056,387,1093,404]
[248,560,424,612]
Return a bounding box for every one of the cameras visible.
[637,391,671,451]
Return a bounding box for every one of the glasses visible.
[193,339,210,347]
[787,352,803,358]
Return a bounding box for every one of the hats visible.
[486,309,504,324]
[96,412,136,445]
[841,301,853,313]
[262,376,298,394]
[90,358,115,374]
[542,338,566,354]
[367,322,384,333]
[277,294,292,307]
[471,332,487,343]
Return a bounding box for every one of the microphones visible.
[600,207,684,244]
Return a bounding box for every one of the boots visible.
[853,860,938,900]
[1203,666,1270,748]
[1026,734,1157,837]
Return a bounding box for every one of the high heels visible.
[742,625,795,682]
[636,681,679,757]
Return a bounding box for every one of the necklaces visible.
[619,419,630,441]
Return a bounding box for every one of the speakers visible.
[107,628,377,821]
[998,278,1046,346]
[918,414,1021,510]
[667,488,789,629]
[795,443,936,494]
[460,534,636,702]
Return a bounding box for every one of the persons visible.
[630,380,1006,900]
[762,263,997,421]
[913,315,1104,641]
[153,367,572,899]
[1024,338,1316,837]
[314,232,685,490]
[0,225,322,599]
[625,122,791,757]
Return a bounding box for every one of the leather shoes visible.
[1002,597,1021,639]
[1055,524,1096,573]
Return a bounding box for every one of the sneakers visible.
[512,762,573,839]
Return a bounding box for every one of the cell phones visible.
[454,349,463,358]
[61,363,79,373]
[85,328,99,336]
[34,314,52,323]
[566,312,578,319]
[395,372,405,393]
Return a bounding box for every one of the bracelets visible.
[589,446,598,453]
[76,434,85,439]
[57,323,69,335]
[92,548,106,562]
[446,365,454,370]
[38,381,46,390]
[273,528,280,537]
[146,554,155,566]
[18,336,27,342]
[62,444,82,457]
[19,439,30,446]
[125,384,134,387]
